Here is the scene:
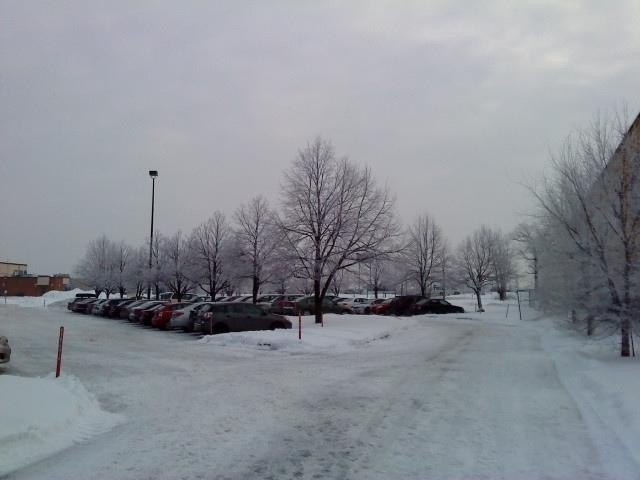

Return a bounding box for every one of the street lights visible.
[146,170,158,299]
